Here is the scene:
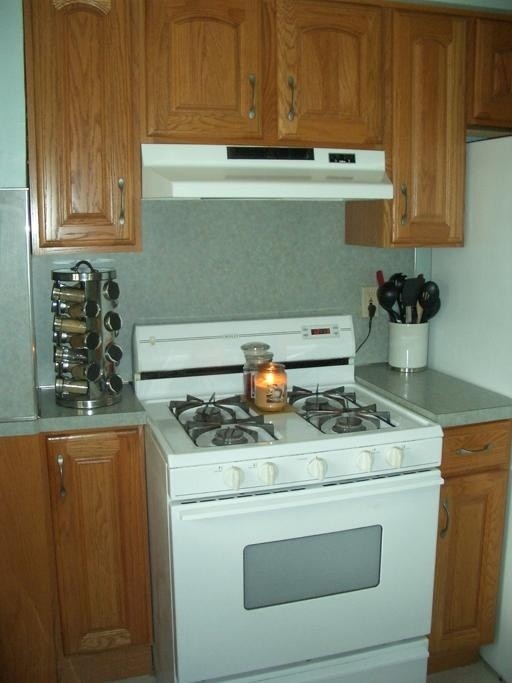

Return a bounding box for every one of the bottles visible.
[48,279,89,399]
[254,362,290,410]
[242,341,274,399]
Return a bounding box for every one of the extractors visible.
[138,144,399,202]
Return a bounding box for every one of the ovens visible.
[146,469,443,682]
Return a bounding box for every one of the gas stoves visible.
[142,377,443,488]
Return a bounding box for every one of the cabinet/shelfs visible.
[143,0,384,152]
[343,0,465,250]
[427,419,512,674]
[39,425,154,657]
[21,1,143,254]
[465,10,512,143]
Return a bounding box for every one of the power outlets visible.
[361,287,379,317]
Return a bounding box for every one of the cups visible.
[386,322,426,370]
